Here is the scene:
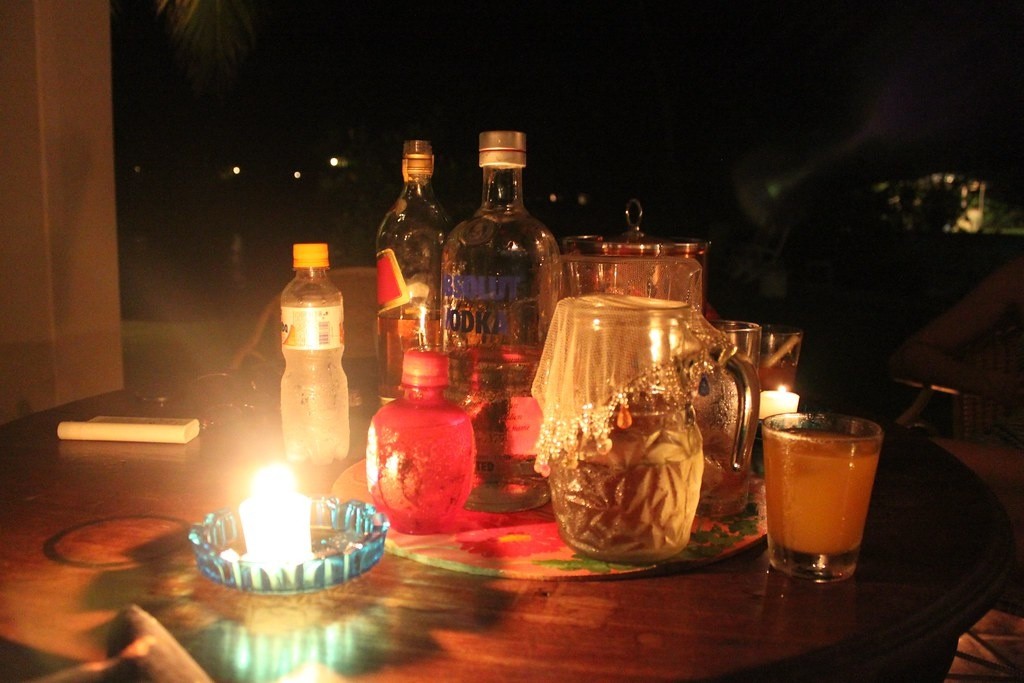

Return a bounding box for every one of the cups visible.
[763,413,885,583]
[691,319,762,518]
[555,254,703,312]
[758,324,803,390]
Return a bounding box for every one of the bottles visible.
[365,351,476,536]
[440,130,564,513]
[558,231,719,318]
[279,242,352,466]
[377,141,448,406]
[457,343,549,494]
[529,295,735,564]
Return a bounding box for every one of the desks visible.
[0,355,1015,683]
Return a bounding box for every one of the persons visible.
[883,254,1023,574]
